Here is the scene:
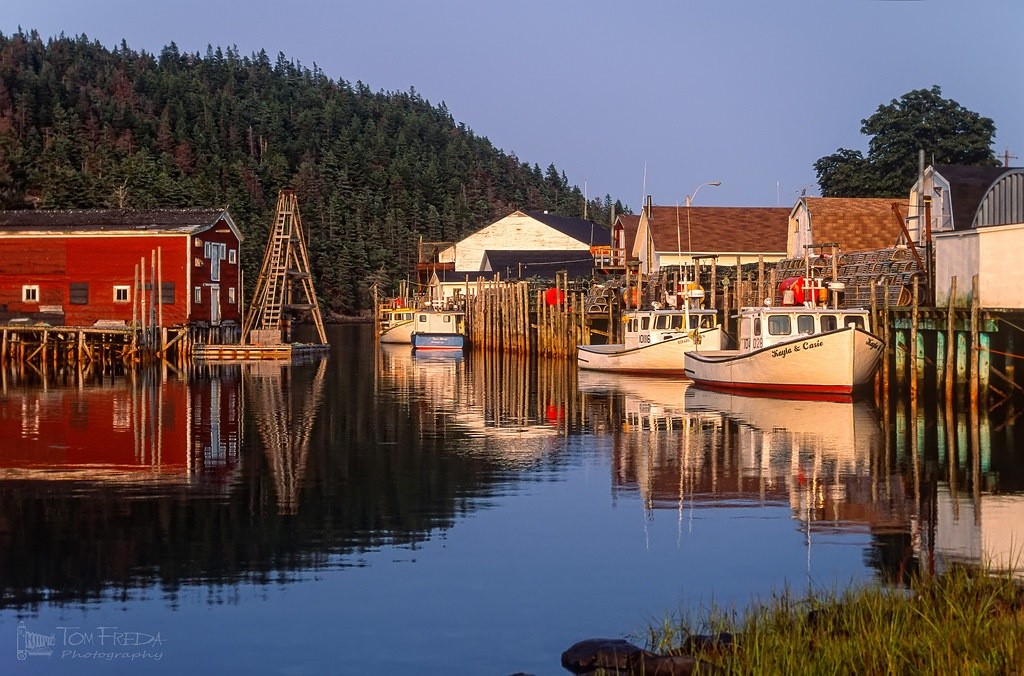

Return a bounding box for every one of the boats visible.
[379,307,469,349]
[575,262,730,376]
[683,200,888,395]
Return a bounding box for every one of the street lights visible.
[687,181,723,252]
